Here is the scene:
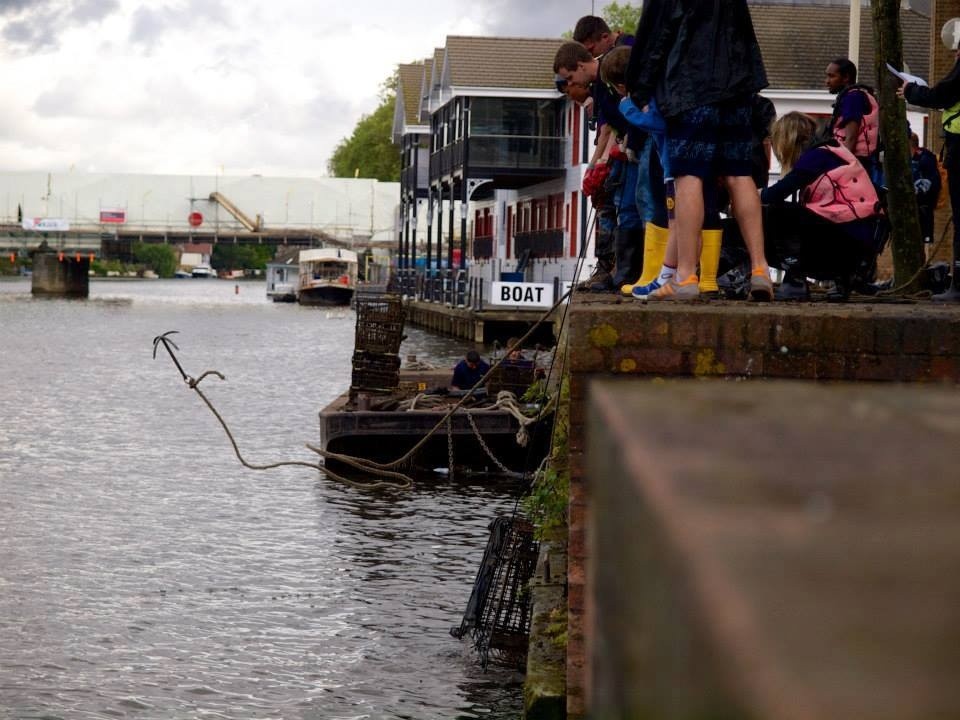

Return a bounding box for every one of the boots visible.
[590,225,643,294]
[698,229,724,293]
[930,260,960,304]
[620,221,670,297]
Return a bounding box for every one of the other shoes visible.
[826,260,868,300]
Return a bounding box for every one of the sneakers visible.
[631,279,661,300]
[648,272,700,298]
[750,267,773,302]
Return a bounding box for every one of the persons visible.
[451,351,490,390]
[757,111,892,301]
[554,15,675,302]
[821,58,884,301]
[866,133,942,295]
[623,0,786,301]
[896,16,960,305]
[500,338,532,368]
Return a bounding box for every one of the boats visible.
[313,342,557,487]
[264,244,360,308]
[174,268,193,279]
[191,262,218,279]
